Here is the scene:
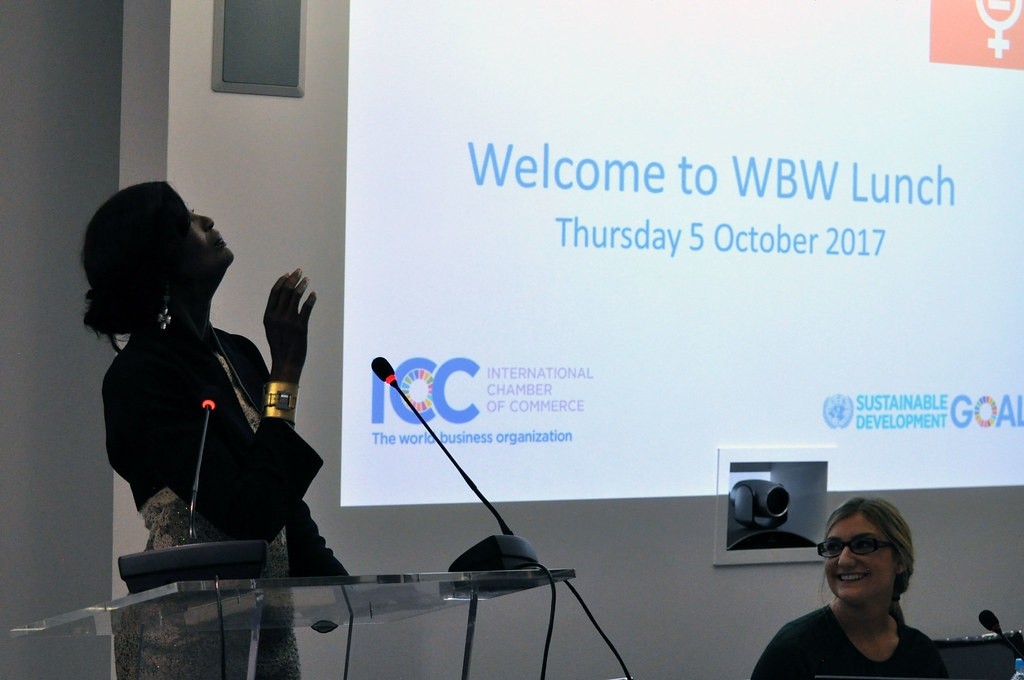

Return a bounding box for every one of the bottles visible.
[1011,658,1024,680]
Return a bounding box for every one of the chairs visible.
[933,630,1024,680]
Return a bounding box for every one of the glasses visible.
[817,536,890,558]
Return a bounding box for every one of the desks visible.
[9,568,577,680]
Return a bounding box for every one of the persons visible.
[81,181,353,680]
[751,495,951,680]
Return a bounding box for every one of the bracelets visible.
[261,381,297,425]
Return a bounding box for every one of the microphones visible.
[371,356,540,601]
[978,609,1024,661]
[119,385,268,594]
